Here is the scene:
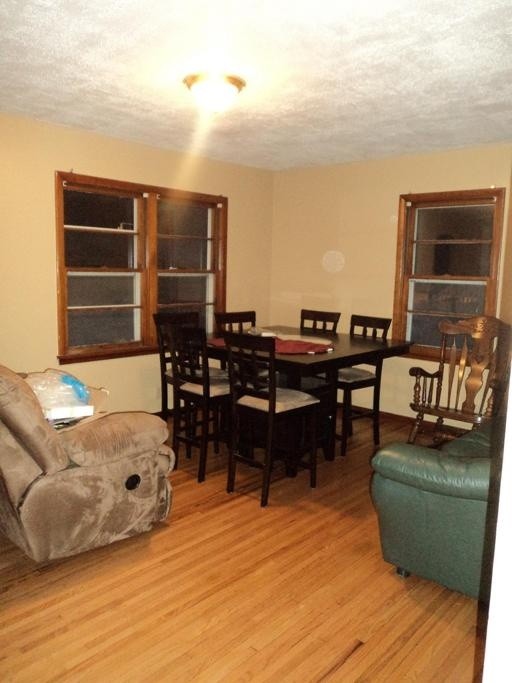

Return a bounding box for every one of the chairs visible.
[300,310,342,380]
[0,365,177,565]
[224,328,321,506]
[315,314,391,457]
[153,311,272,484]
[407,313,512,448]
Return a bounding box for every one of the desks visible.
[170,324,415,478]
